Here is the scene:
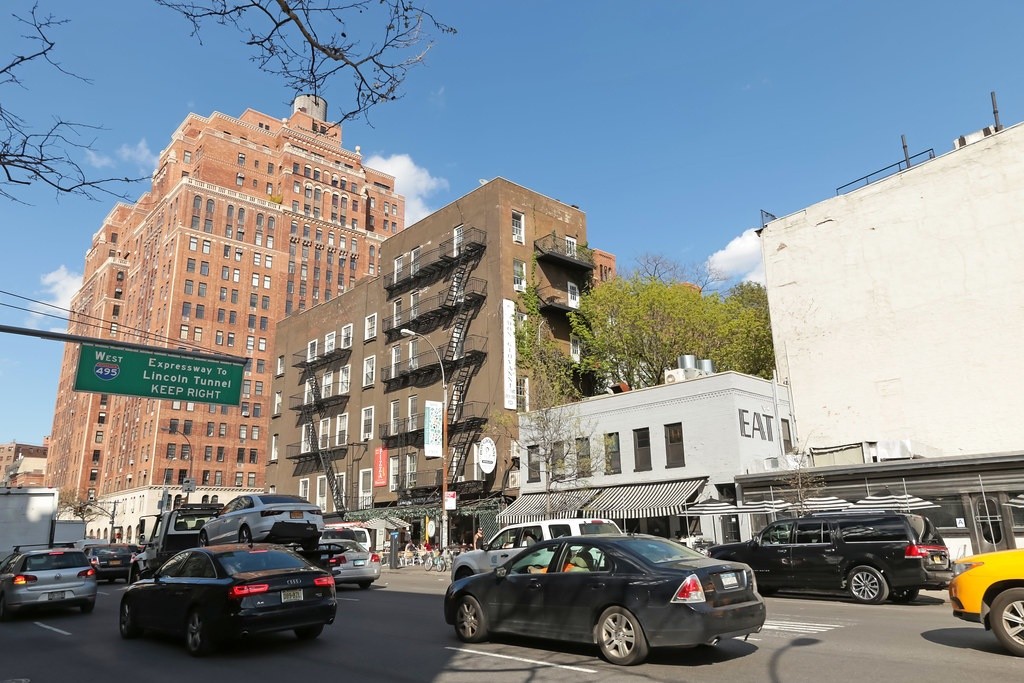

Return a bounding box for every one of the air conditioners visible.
[665,369,685,383]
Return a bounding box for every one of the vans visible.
[319,525,372,552]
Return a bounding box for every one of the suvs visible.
[449,517,624,583]
[706,513,954,605]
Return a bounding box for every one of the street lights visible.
[160,457,178,516]
[400,328,448,564]
[161,426,195,523]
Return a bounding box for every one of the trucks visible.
[129,502,350,582]
[0,485,59,563]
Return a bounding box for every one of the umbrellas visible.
[361,516,411,554]
[842,485,941,512]
[726,494,792,525]
[785,490,855,513]
[676,495,739,546]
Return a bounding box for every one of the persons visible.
[523,528,538,546]
[405,537,432,565]
[527,546,575,573]
[473,526,484,552]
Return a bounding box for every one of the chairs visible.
[177,521,188,530]
[569,552,594,572]
[379,550,439,566]
[527,535,537,547]
[192,520,205,529]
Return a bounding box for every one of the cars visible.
[0,519,138,622]
[120,543,338,657]
[199,493,325,551]
[950,548,1024,658]
[443,530,767,667]
[295,538,381,589]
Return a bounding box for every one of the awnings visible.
[494,488,600,525]
[581,479,706,520]
